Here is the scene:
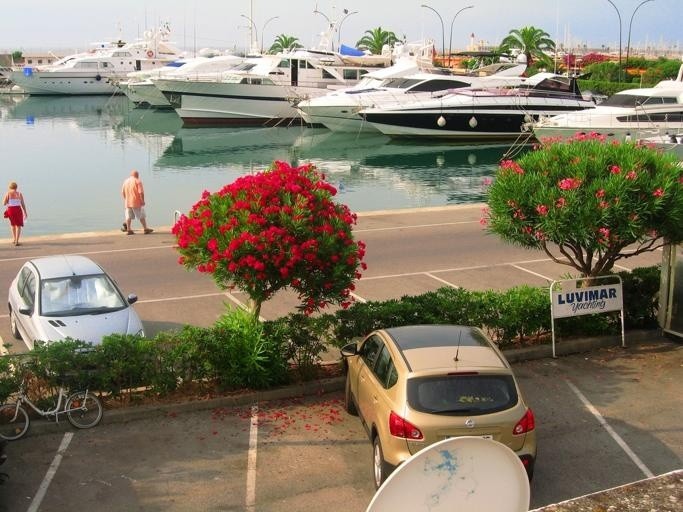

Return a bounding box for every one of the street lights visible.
[420,3,445,66]
[261,16,280,54]
[449,5,476,66]
[338,12,359,52]
[313,10,334,51]
[240,13,258,51]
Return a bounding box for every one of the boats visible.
[0,37,244,110]
[637,131,682,162]
[355,136,525,203]
[527,64,683,165]
[2,94,182,136]
[294,54,532,137]
[290,125,391,169]
[153,124,294,168]
[356,73,609,140]
[149,43,356,129]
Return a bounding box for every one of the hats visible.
[130,171,138,176]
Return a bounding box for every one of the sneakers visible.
[143,229,154,233]
[13,240,18,246]
[127,230,133,234]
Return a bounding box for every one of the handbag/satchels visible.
[4,210,8,218]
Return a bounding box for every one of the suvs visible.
[339,323,538,492]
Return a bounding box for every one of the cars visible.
[7,253,146,380]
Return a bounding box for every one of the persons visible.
[3,182,28,245]
[122,171,154,235]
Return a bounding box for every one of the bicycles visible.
[0,361,104,440]
